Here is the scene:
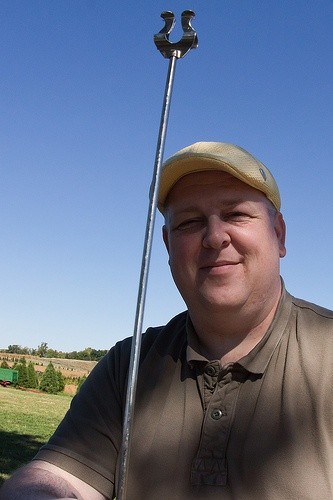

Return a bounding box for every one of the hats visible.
[150,140,280,213]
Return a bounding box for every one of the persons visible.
[1,141,333,500]
